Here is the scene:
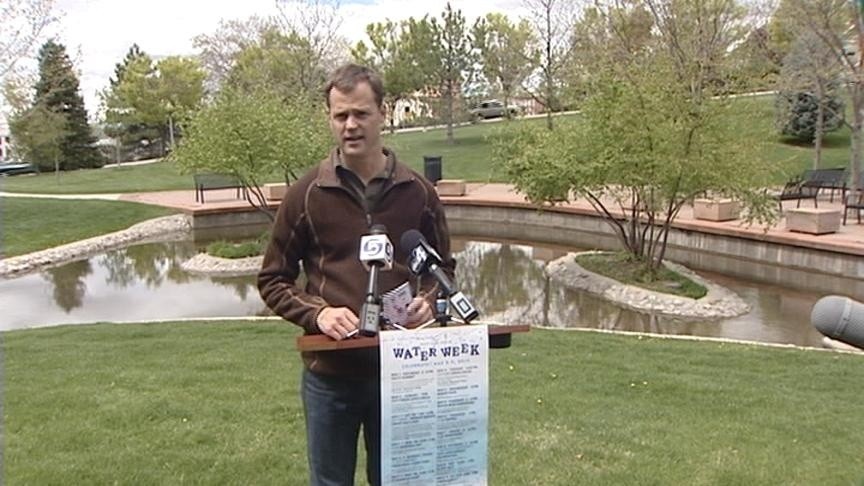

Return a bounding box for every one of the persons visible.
[256,59,455,486]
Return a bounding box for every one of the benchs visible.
[765,165,864,224]
[192,169,248,204]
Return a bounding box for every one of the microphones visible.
[399,230,478,324]
[356,224,395,335]
[810,295,864,352]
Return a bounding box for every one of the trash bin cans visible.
[423,155,442,186]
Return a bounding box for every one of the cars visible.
[469,98,517,121]
